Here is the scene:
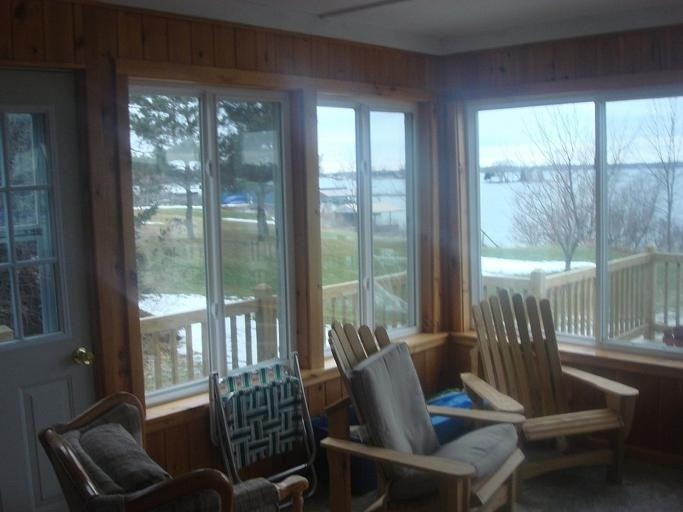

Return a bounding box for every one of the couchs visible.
[38,391,310,512]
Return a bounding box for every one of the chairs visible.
[321,318,525,512]
[462,290,640,500]
[209,351,319,496]
